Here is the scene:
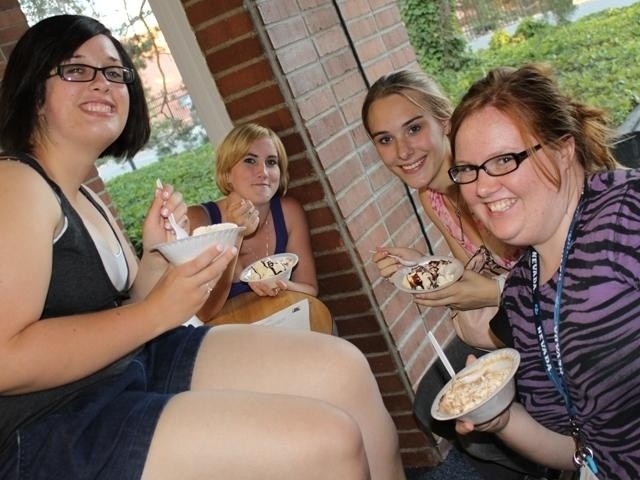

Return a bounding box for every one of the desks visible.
[205,288,340,335]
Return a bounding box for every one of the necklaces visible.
[447,191,465,241]
[242,217,269,260]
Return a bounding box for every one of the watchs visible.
[573,444,593,476]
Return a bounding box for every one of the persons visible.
[445,64,640,479]
[361,70,565,479]
[186,122,338,336]
[0,14,407,480]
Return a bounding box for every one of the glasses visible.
[47,64,136,84]
[448,143,547,185]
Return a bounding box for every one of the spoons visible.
[155,177,188,241]
[369,250,418,267]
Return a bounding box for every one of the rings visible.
[204,283,213,292]
[247,210,252,216]
